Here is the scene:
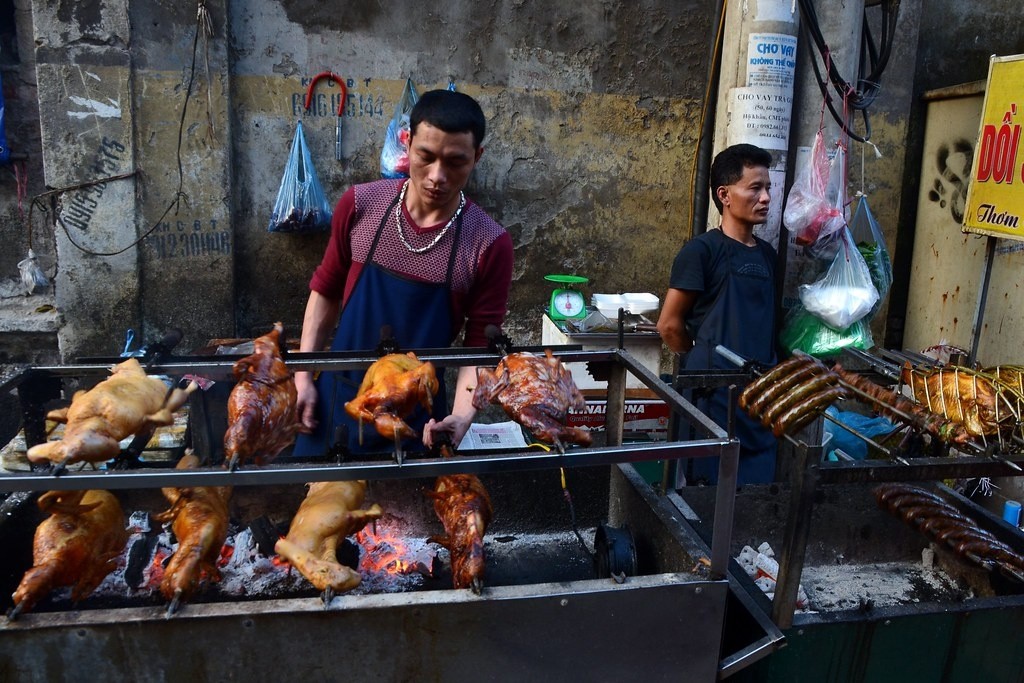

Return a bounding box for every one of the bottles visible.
[1003,501,1021,528]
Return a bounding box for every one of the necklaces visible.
[396,178,466,254]
[752,238,755,241]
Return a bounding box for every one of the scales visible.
[544,275,589,321]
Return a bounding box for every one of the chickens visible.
[12,321,592,626]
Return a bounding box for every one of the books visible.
[458,420,528,450]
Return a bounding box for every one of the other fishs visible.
[898,360,1015,438]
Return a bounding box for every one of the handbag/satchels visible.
[823,144,851,221]
[798,225,880,332]
[783,130,846,241]
[824,197,894,324]
[378,79,417,177]
[266,122,333,234]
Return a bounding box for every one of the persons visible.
[287,89,515,461]
[482,434,500,443]
[656,144,785,489]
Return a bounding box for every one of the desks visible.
[542,313,660,389]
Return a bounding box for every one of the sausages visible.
[738,354,847,438]
[878,484,1024,581]
